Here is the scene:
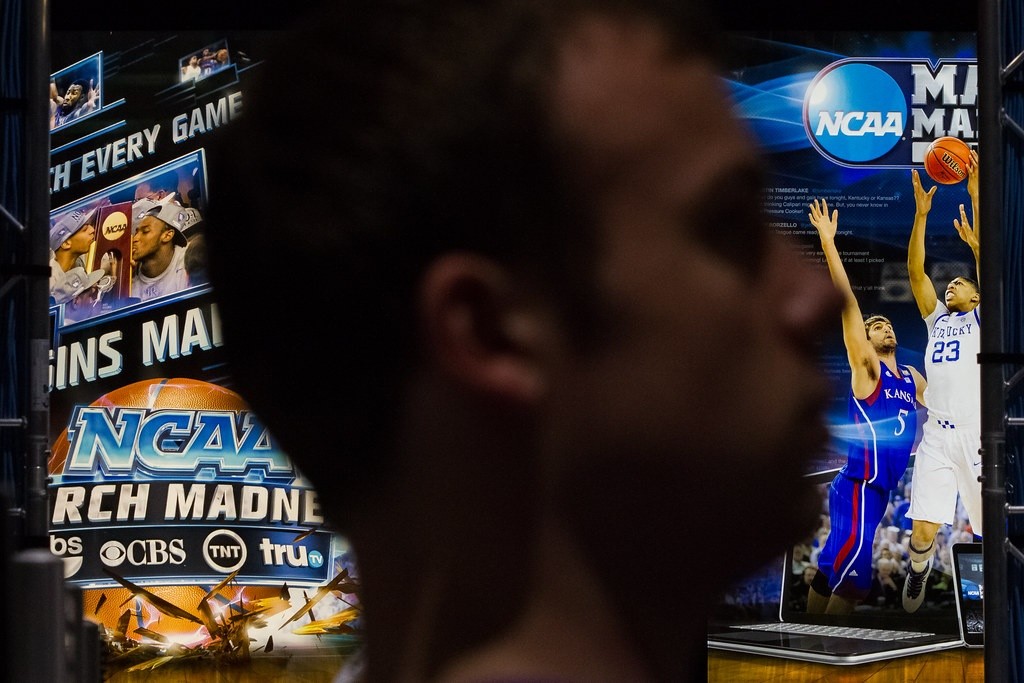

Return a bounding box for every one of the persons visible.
[902,149,982,614]
[180,48,228,83]
[48,161,207,325]
[203,0,846,682]
[792,480,972,615]
[50,77,99,128]
[805,196,929,624]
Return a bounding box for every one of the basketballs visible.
[924,136,971,185]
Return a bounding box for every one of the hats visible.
[131,192,176,229]
[145,202,187,247]
[50,206,95,251]
[53,267,106,305]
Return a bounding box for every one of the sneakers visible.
[902,554,934,612]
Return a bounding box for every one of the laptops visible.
[704,450,962,665]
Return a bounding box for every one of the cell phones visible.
[950,542,984,649]
